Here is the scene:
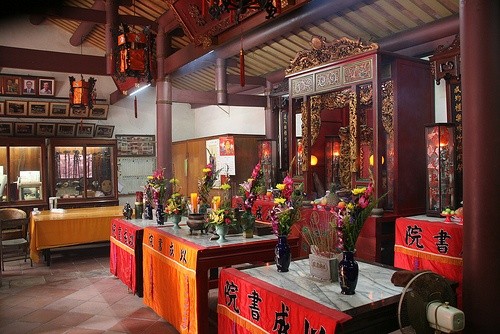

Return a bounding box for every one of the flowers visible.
[145,149,394,252]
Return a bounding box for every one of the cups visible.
[33,208,38,212]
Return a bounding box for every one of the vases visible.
[216,223,228,244]
[171,214,181,231]
[339,251,358,294]
[152,207,157,221]
[273,236,291,272]
[242,221,253,239]
[145,203,151,219]
[156,204,164,226]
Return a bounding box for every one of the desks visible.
[29,206,135,266]
[219,254,461,334]
[109,216,189,293]
[142,225,301,334]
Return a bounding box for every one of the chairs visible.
[0,208,28,270]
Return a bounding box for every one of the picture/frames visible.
[0,122,114,139]
[0,73,55,97]
[0,100,109,120]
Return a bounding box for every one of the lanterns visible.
[69,76,96,127]
[208,0,277,87]
[112,22,153,118]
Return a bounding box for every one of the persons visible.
[41,82,52,94]
[24,81,34,93]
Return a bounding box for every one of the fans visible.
[398,271,465,334]
